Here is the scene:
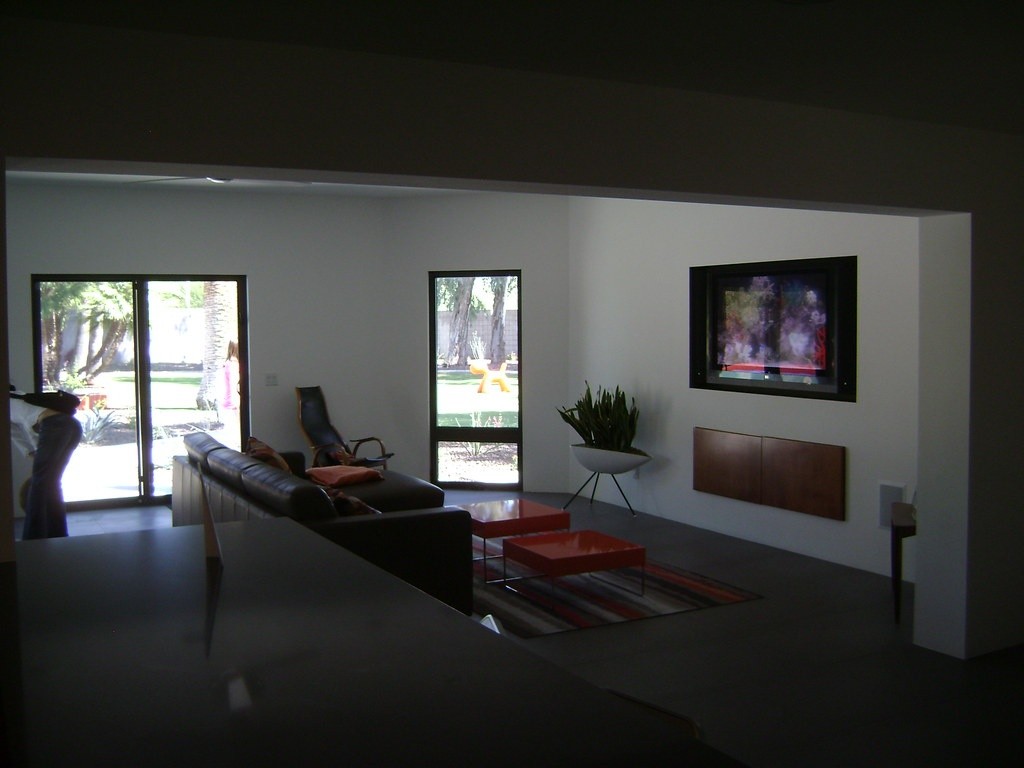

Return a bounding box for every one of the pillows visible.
[317,485,383,516]
[246,436,291,474]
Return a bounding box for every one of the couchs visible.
[172,432,473,606]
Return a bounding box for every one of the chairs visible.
[295,385,394,470]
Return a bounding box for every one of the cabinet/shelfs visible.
[16,516,748,768]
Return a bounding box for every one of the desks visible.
[891,502,916,624]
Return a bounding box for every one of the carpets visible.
[472,530,764,638]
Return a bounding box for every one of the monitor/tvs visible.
[707,264,841,385]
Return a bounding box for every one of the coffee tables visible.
[503,531,646,610]
[461,499,571,585]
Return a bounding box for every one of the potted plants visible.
[555,380,652,475]
